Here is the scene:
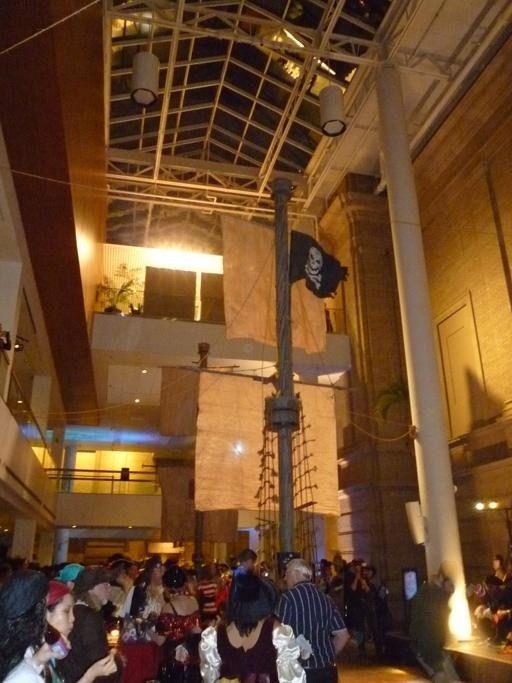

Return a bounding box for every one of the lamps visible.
[130,50,159,107]
[319,39,348,136]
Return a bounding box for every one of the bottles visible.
[44,620,70,660]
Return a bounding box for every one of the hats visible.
[0,563,121,620]
[106,553,132,568]
[163,566,187,589]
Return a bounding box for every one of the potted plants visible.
[97,261,144,314]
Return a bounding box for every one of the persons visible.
[1,547,511,682]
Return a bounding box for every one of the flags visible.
[287,226,352,299]
